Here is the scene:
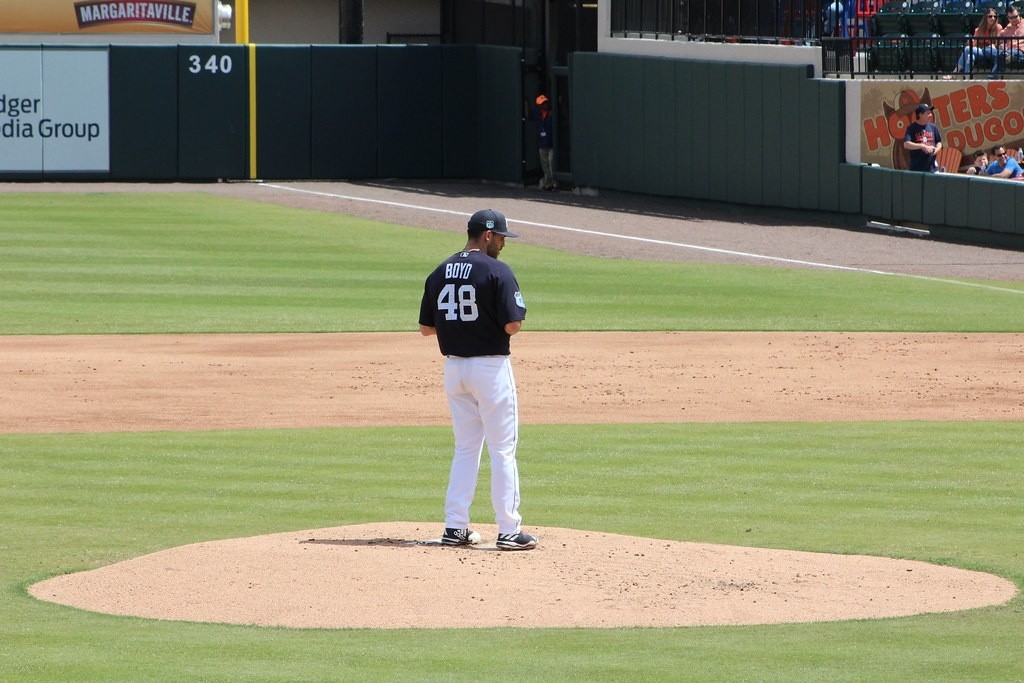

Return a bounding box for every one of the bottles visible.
[981,163,986,175]
[1017,147,1023,161]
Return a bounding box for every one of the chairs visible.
[871,0,1024,73]
[937,147,963,173]
[990,148,1019,164]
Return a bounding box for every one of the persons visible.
[966,149,989,175]
[524,95,560,192]
[418,209,539,549]
[982,5,1024,79]
[979,145,1023,178]
[942,8,1002,81]
[904,104,943,173]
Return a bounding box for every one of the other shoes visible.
[943,75,957,80]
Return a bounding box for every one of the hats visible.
[535,95,548,104]
[915,104,935,111]
[468,208,519,238]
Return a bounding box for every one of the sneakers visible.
[441,528,481,544]
[496,533,537,550]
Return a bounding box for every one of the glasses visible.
[995,151,1006,157]
[1007,14,1019,19]
[986,14,996,18]
